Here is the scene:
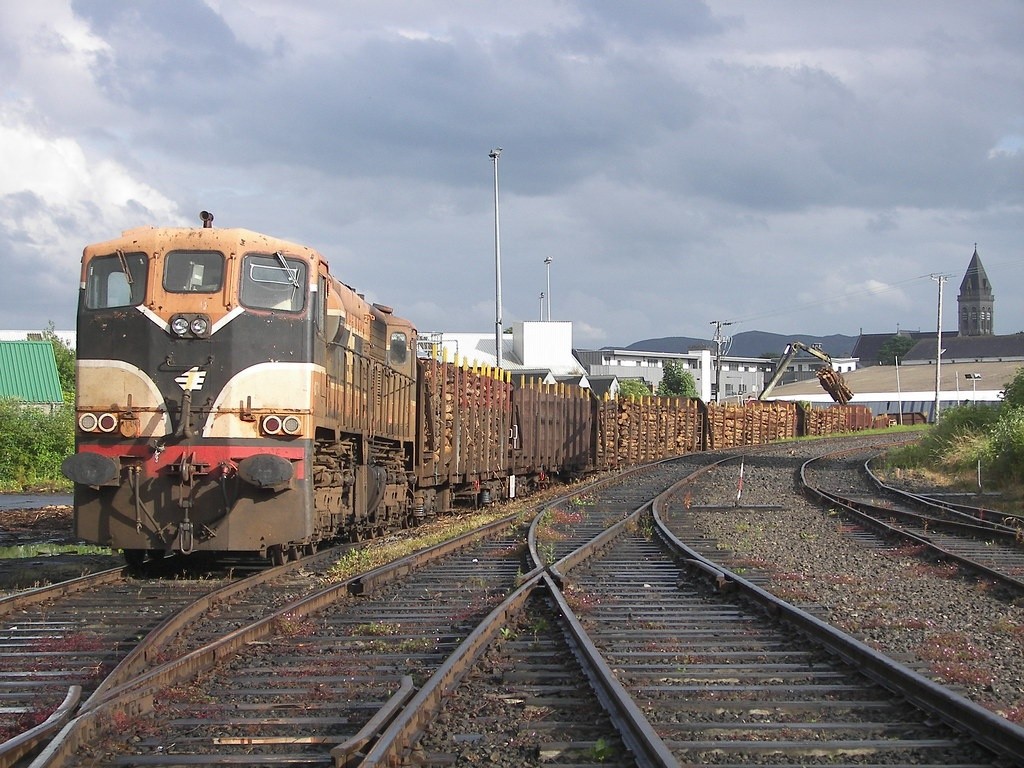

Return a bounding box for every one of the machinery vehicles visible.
[759,340,835,400]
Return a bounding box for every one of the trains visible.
[62,208,930,565]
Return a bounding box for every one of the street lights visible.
[966,373,982,405]
[489,146,502,366]
[543,257,552,322]
[538,293,542,322]
